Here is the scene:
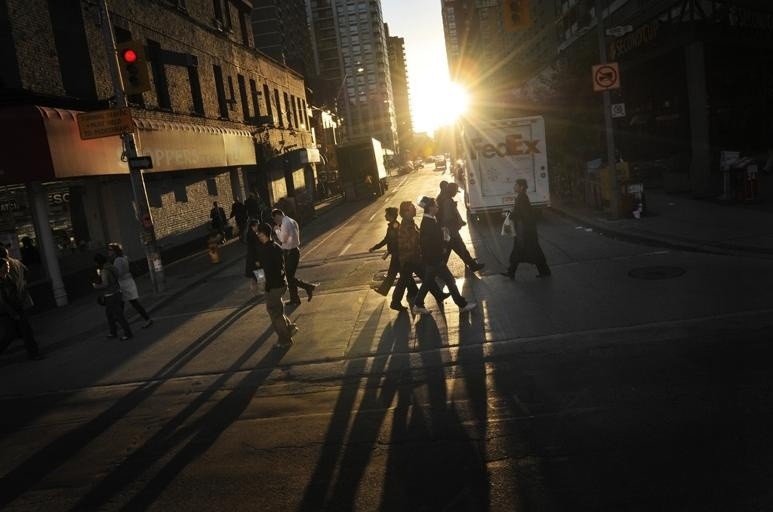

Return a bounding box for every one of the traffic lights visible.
[116,37,151,94]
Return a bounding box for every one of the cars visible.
[402,154,463,177]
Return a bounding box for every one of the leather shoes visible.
[105,333,115,337]
[141,320,153,328]
[500,271,514,279]
[119,335,128,341]
[285,298,301,306]
[306,284,314,302]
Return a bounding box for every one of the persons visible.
[91,254,134,342]
[211,192,319,351]
[109,241,154,329]
[370,179,487,313]
[0,247,35,312]
[500,178,551,279]
[21,236,44,280]
[0,259,43,361]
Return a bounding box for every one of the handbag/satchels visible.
[252,266,266,295]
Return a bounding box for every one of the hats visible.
[418,197,432,209]
[446,183,459,192]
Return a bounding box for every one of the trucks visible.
[457,113,552,219]
[334,136,389,202]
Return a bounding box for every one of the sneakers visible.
[289,325,298,337]
[372,263,484,315]
[272,337,293,348]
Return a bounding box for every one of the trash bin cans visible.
[620,177,649,219]
